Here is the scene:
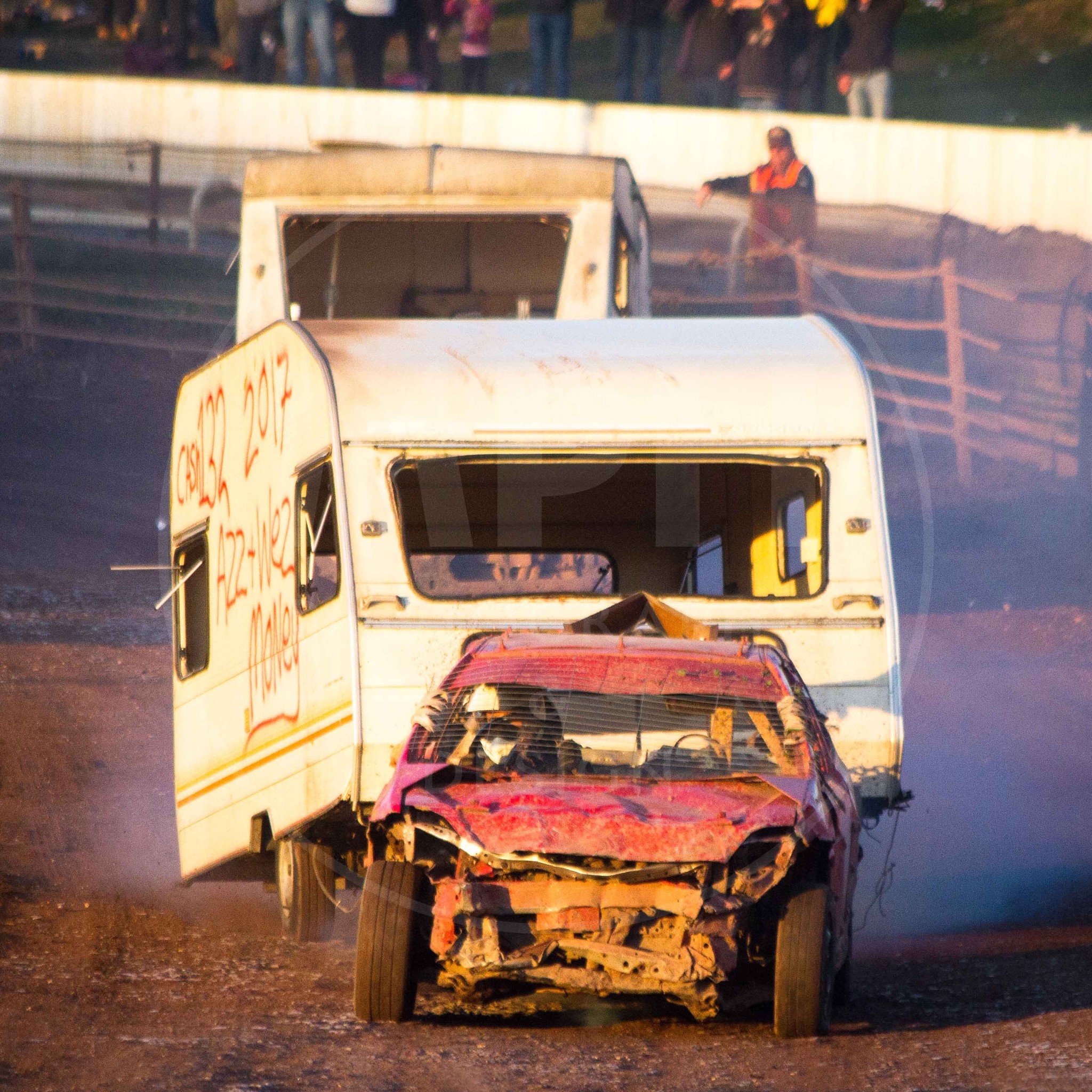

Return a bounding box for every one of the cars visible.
[328,593,867,1031]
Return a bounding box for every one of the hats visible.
[767,127,791,148]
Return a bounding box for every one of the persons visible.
[447,688,581,778]
[677,0,907,117]
[0,0,337,86]
[605,0,672,104]
[529,0,572,98]
[698,126,817,316]
[330,0,492,94]
